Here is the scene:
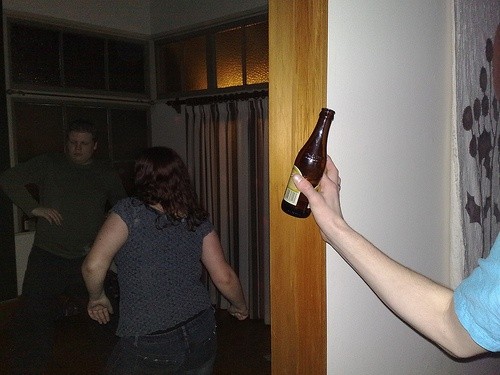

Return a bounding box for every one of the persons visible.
[0,118,141,375]
[76,146,251,374]
[291,23,500,361]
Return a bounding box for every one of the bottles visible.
[281,107,335,219]
